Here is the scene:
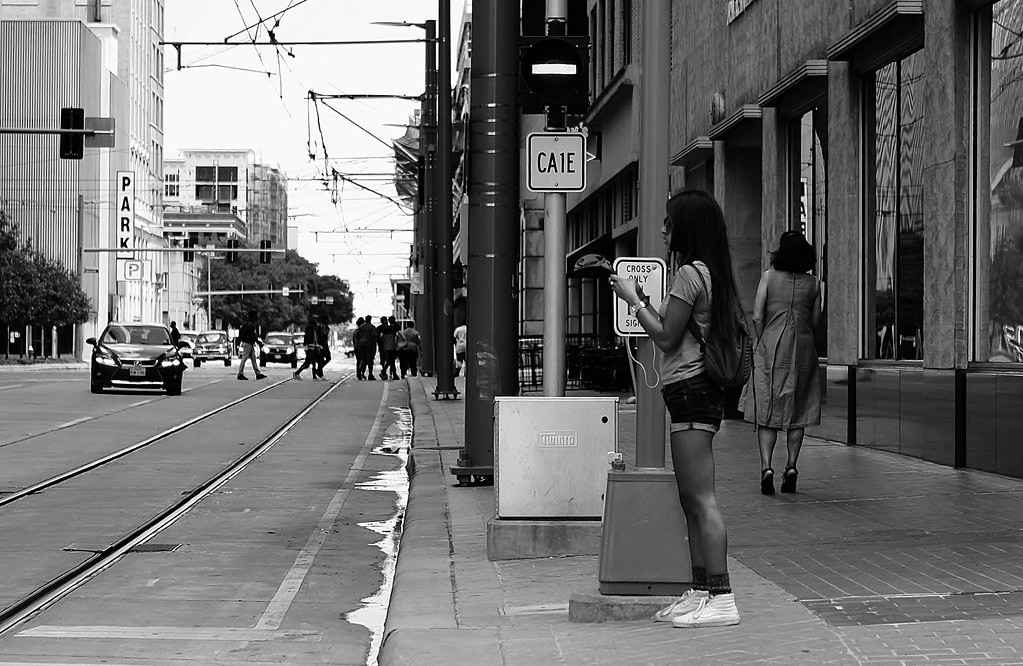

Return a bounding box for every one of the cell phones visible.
[607,262,616,275]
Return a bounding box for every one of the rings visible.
[610,282,614,286]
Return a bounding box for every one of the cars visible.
[294,332,308,359]
[86,321,190,397]
[259,331,299,368]
[237,341,260,358]
[344,347,355,359]
[191,331,232,367]
[178,331,200,354]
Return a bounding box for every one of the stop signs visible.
[520,35,589,107]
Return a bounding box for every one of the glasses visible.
[663,216,672,230]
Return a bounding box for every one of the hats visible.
[312,314,319,317]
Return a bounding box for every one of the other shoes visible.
[356,371,407,380]
[297,374,302,380]
[237,373,248,380]
[314,377,321,381]
[256,373,267,380]
[293,372,297,379]
[321,376,329,380]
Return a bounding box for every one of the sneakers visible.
[671,593,740,627]
[652,588,709,622]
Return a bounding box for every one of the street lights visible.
[374,19,434,378]
[76,200,108,362]
[168,235,190,333]
[140,224,163,323]
[208,256,227,329]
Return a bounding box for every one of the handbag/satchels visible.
[396,331,407,351]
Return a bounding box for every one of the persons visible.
[744,230,821,494]
[169,321,181,344]
[453,319,466,377]
[292,314,423,381]
[608,187,741,627]
[237,311,267,381]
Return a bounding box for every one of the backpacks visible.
[683,258,752,385]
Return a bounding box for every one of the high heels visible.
[761,468,775,496]
[780,465,799,494]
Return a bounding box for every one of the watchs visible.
[628,301,646,317]
